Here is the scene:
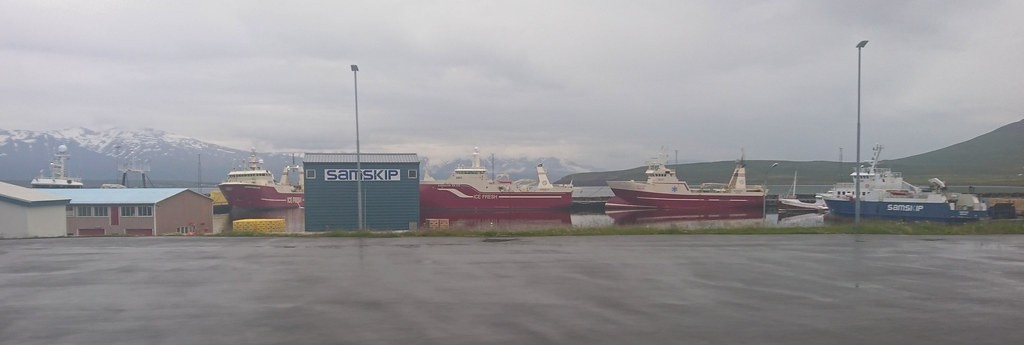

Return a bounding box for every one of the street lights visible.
[839,147,844,180]
[855,39,869,228]
[350,65,363,231]
[763,163,778,224]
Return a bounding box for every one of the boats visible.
[777,169,832,213]
[29,144,83,188]
[218,144,305,209]
[420,146,576,207]
[605,209,767,228]
[604,145,768,207]
[823,144,986,224]
[419,209,573,228]
